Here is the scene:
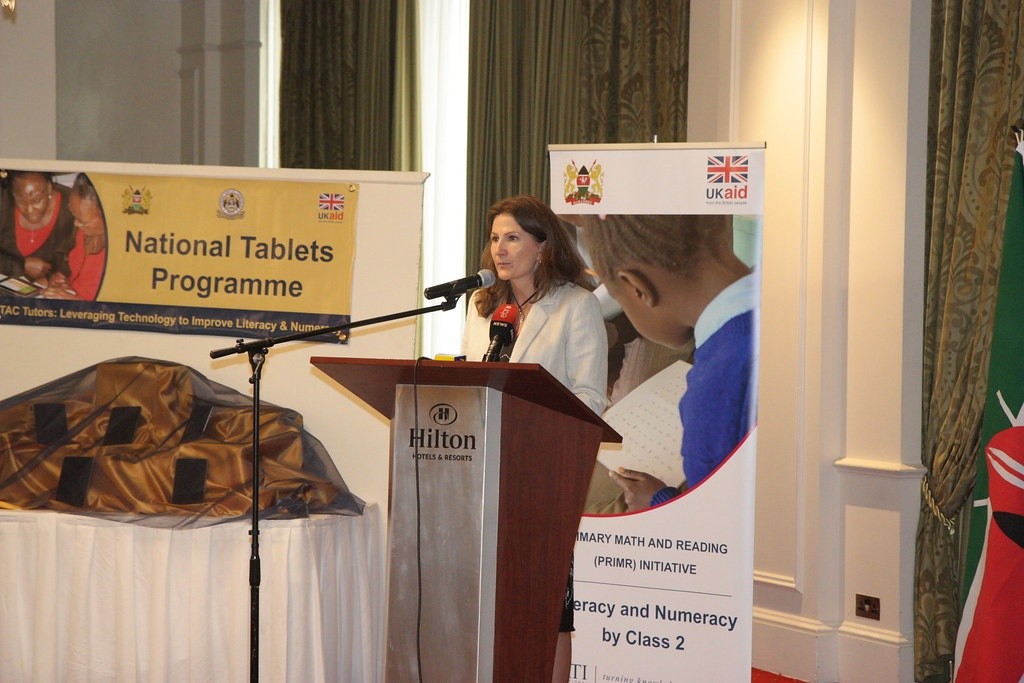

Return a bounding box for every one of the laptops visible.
[33,402,81,446]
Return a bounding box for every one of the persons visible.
[0,170,107,300]
[584,201,753,510]
[475,196,609,417]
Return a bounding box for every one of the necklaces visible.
[30,221,48,243]
[512,290,539,324]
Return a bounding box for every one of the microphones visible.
[483,304,522,362]
[424,269,496,300]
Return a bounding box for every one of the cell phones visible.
[0,276,42,298]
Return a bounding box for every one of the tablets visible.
[57,405,213,508]
